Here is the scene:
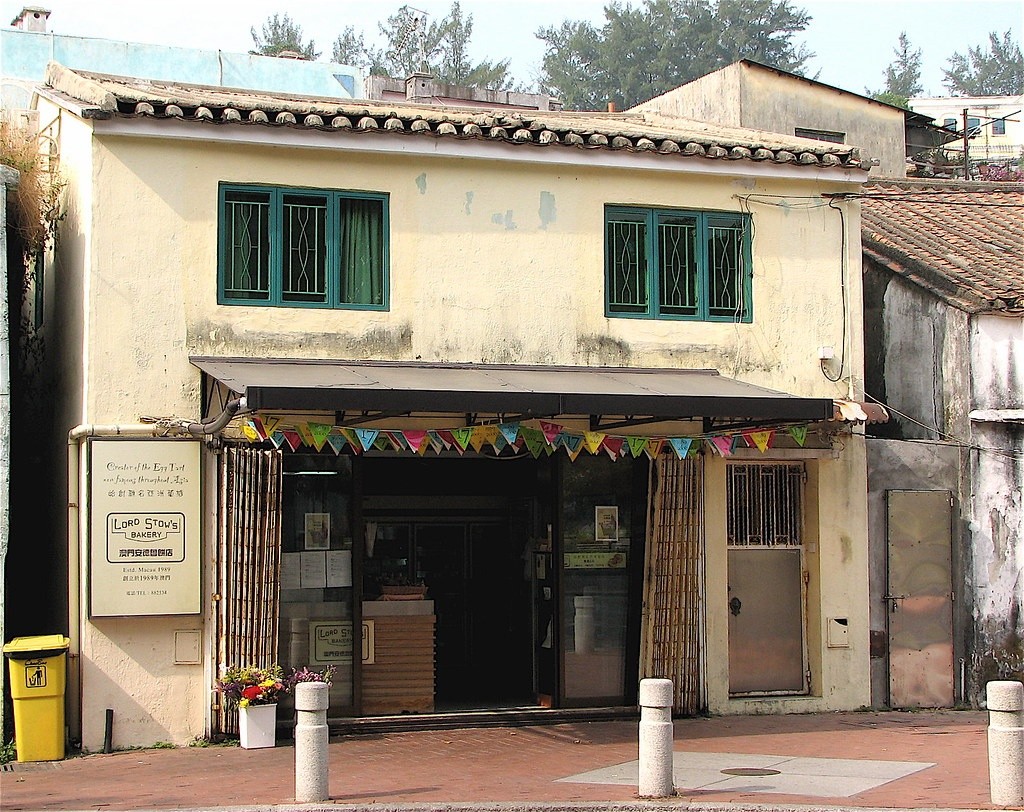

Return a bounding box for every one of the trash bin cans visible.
[2,634,71,763]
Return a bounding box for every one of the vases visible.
[237,703,278,749]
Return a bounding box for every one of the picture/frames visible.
[172,629,202,665]
[595,506,619,541]
[305,513,330,550]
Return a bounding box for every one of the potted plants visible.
[378,575,429,595]
[913,144,1000,177]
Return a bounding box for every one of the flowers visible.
[211,664,288,717]
[285,664,338,697]
